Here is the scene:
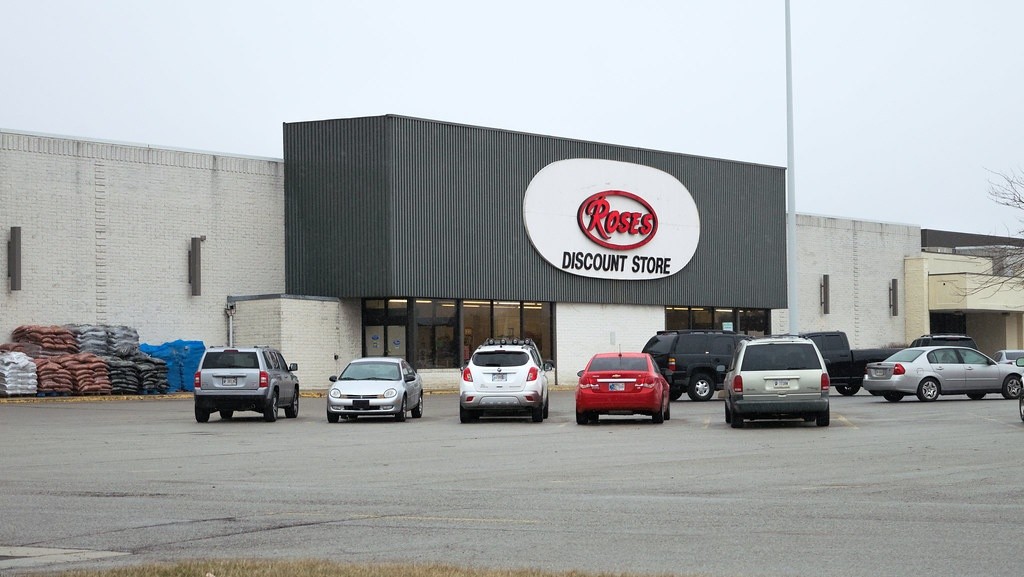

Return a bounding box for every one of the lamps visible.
[820,274,830,313]
[890,278,899,317]
[7,227,22,290]
[188,237,201,297]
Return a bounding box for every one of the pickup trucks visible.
[774,330,903,395]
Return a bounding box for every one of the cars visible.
[863,345,1024,402]
[991,350,1024,367]
[326,356,424,422]
[575,353,671,425]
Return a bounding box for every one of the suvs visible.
[193,344,300,422]
[457,337,554,423]
[641,330,753,401]
[909,333,979,353]
[717,334,831,427]
